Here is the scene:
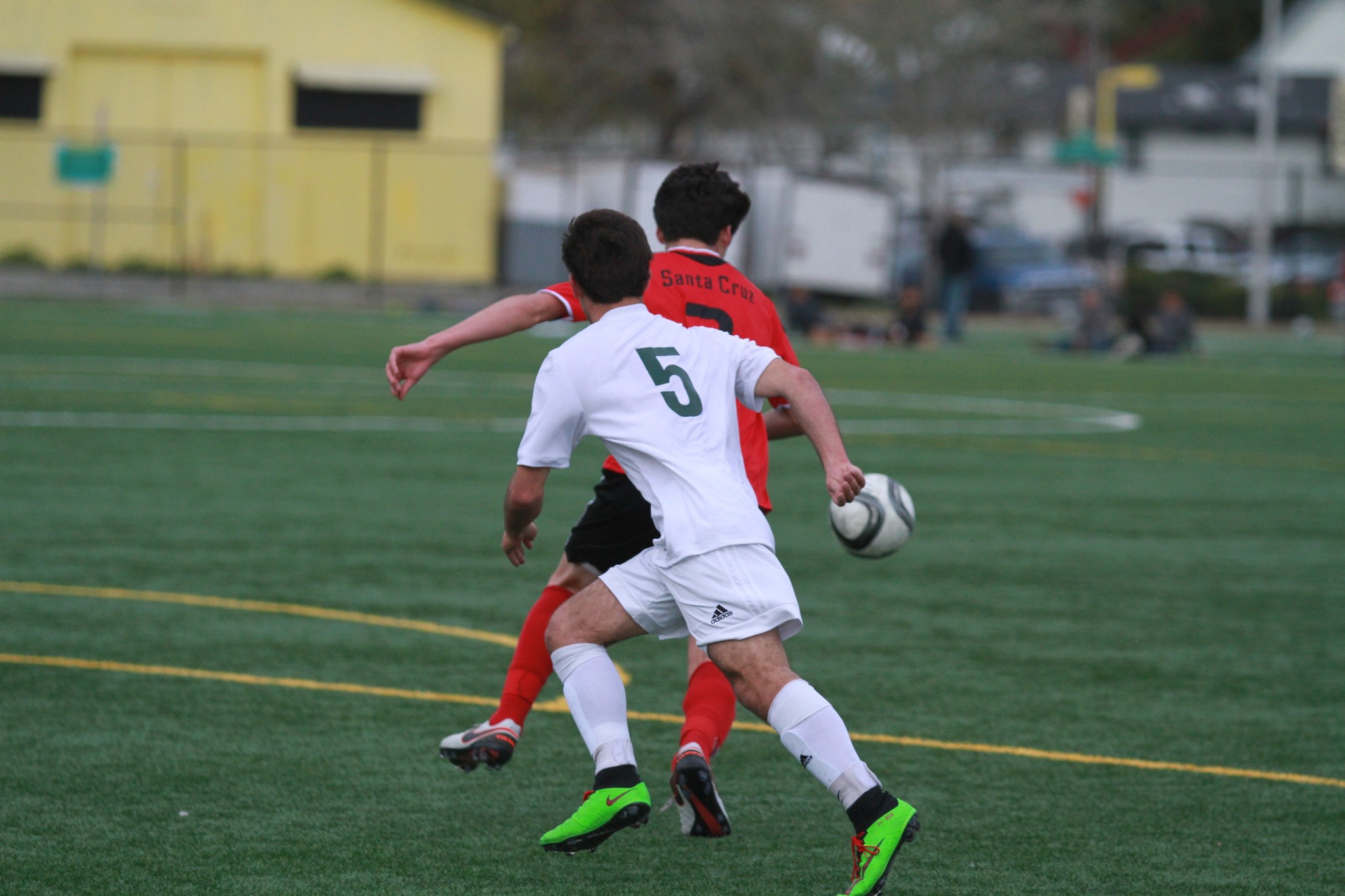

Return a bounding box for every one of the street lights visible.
[1091,62,1165,210]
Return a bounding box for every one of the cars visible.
[779,211,1114,320]
[1057,211,1345,321]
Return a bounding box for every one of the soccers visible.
[827,472,915,560]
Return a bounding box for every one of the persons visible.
[938,198,979,344]
[498,206,924,896]
[385,160,804,838]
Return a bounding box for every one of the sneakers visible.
[439,719,522,774]
[540,782,651,855]
[667,747,732,838]
[838,795,920,896]
